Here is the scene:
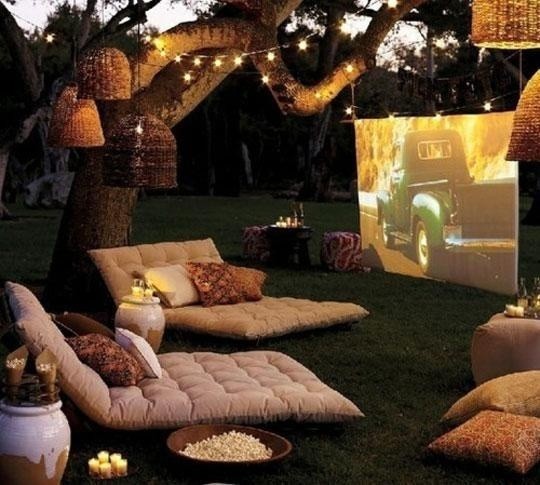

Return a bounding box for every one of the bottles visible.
[531,277,540,308]
[517,278,528,308]
[290,202,304,226]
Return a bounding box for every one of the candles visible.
[88,449,127,478]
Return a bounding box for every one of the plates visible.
[165,422,294,465]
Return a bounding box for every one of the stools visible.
[241,222,271,267]
[321,228,362,275]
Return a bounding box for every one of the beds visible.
[0,276,373,438]
[73,235,371,347]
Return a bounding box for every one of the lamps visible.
[469,0,539,164]
[47,0,182,191]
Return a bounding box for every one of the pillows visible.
[53,307,164,388]
[131,255,265,308]
[425,366,539,475]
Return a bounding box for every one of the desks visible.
[468,309,540,386]
[267,224,312,270]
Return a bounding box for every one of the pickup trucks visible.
[374,130,520,278]
